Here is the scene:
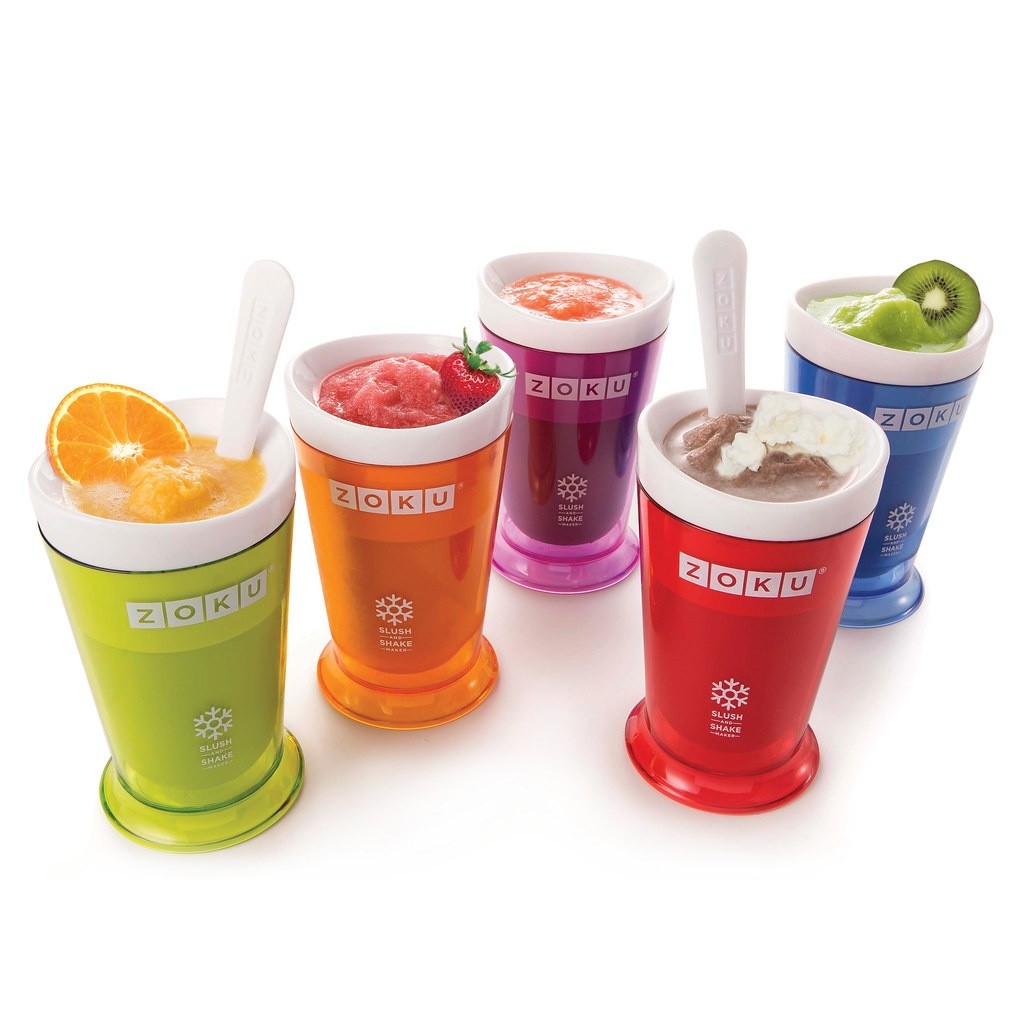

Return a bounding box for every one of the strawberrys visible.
[440,326,519,416]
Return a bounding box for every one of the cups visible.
[784,275,994,628]
[474,249,677,597]
[284,332,501,729]
[625,386,892,816]
[28,394,310,852]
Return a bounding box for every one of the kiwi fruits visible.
[892,260,981,339]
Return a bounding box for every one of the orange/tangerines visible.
[45,383,192,487]
